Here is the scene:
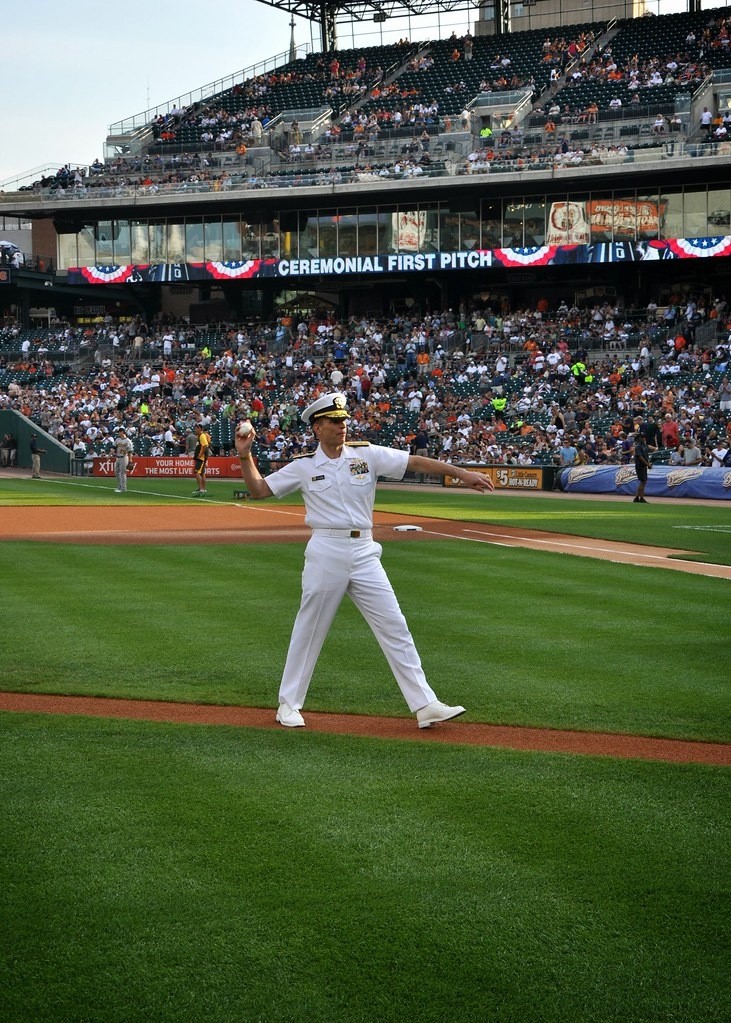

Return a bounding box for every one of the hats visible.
[31,434,37,437]
[301,393,351,424]
[117,428,125,433]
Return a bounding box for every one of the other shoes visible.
[193,489,206,494]
[634,498,639,502]
[32,476,36,478]
[37,477,40,479]
[114,489,121,492]
[639,498,647,502]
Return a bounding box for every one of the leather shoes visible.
[417,701,466,729]
[275,703,306,727]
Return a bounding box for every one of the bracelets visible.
[239,452,252,460]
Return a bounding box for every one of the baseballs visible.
[239,422,254,438]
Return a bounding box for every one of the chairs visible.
[0,327,731,466]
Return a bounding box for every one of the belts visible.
[313,529,373,538]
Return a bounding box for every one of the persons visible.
[632,434,653,503]
[234,391,496,728]
[480,54,535,95]
[0,295,731,474]
[31,433,47,479]
[191,423,209,496]
[631,94,640,103]
[566,43,713,90]
[444,94,628,175]
[539,29,595,94]
[686,16,731,47]
[235,58,418,102]
[34,101,440,202]
[654,113,681,134]
[700,106,713,134]
[399,36,408,45]
[449,30,473,61]
[711,111,731,141]
[407,54,434,71]
[112,428,133,493]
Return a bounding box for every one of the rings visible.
[485,474,489,478]
